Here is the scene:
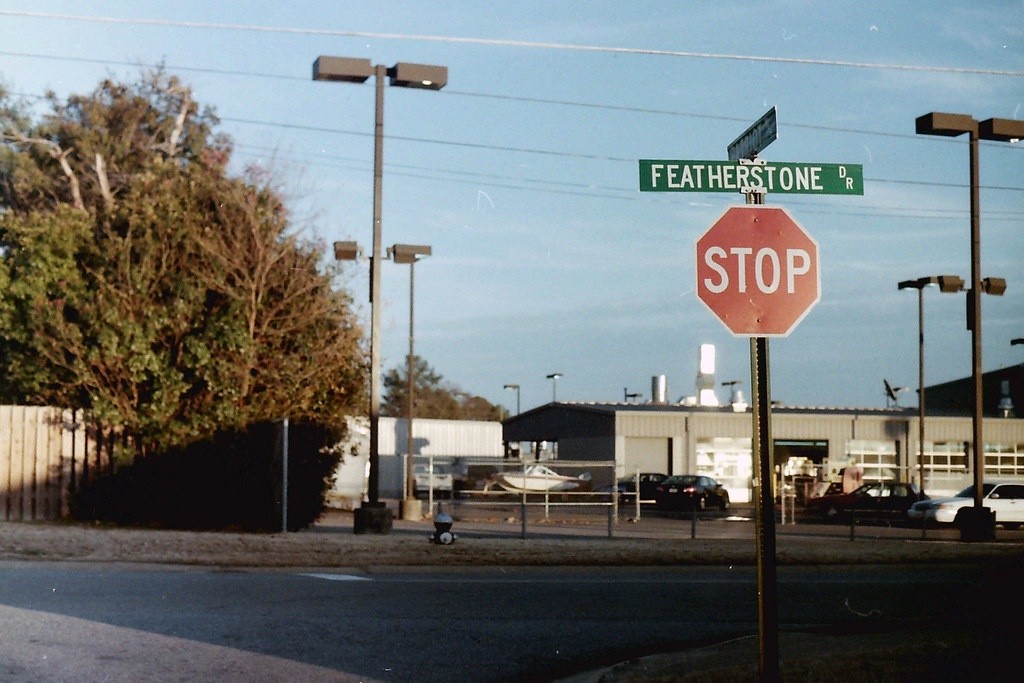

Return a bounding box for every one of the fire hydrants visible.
[429,512,458,545]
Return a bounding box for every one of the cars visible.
[806,481,930,523]
[596,472,667,505]
[906,479,1024,528]
[655,475,729,514]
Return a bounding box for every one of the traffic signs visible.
[727,106,778,159]
[639,158,865,195]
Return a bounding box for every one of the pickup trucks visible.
[411,463,452,500]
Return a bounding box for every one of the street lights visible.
[900,276,941,540]
[313,56,445,512]
[624,392,641,403]
[504,385,520,415]
[546,373,562,402]
[722,380,744,396]
[331,241,433,501]
[915,112,1024,513]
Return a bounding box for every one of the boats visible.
[490,466,590,493]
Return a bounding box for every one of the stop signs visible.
[695,203,820,337]
